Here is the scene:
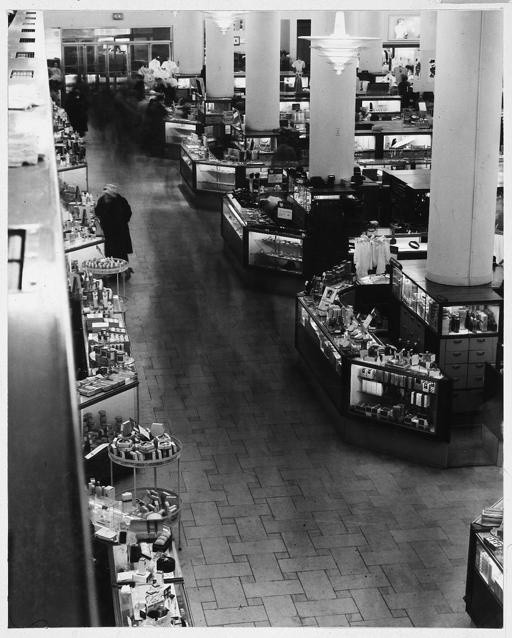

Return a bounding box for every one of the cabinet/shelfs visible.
[351,349,447,436]
[80,258,128,328]
[105,432,184,552]
[117,68,434,283]
[296,295,384,418]
[297,265,378,312]
[390,259,501,391]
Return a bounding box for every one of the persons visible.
[93,183,135,283]
[48,62,66,94]
[149,55,161,76]
[138,62,154,87]
[64,88,92,137]
[291,57,306,74]
[397,74,412,109]
[142,93,173,136]
[160,56,180,79]
[282,48,287,57]
